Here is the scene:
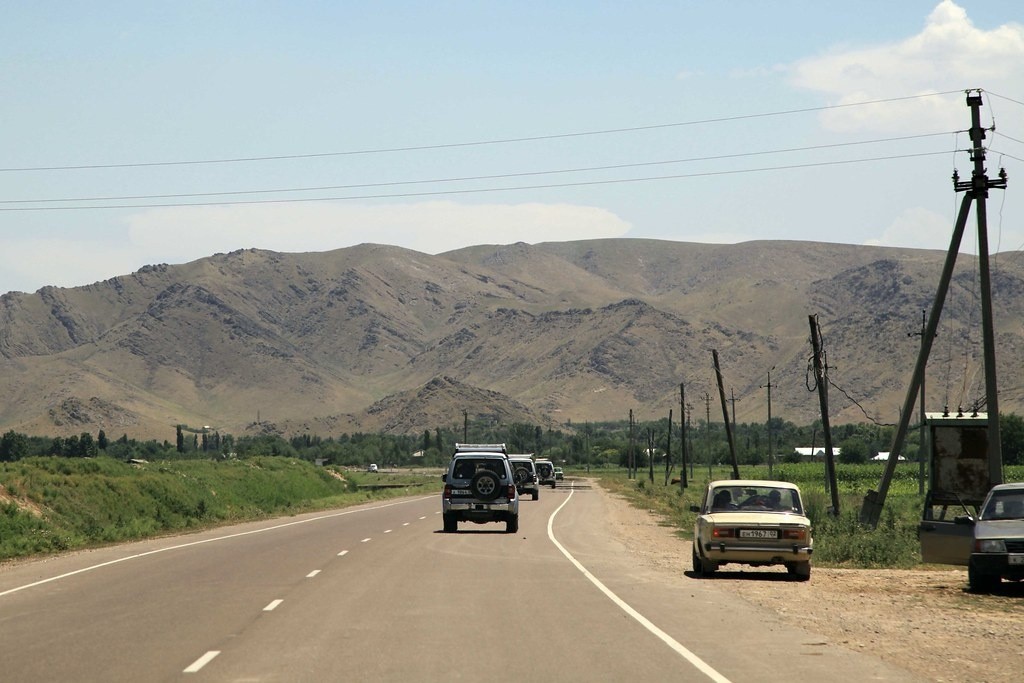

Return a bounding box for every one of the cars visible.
[693,479,815,582]
[970,482,1024,593]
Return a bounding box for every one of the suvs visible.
[439,442,520,533]
[507,454,540,503]
[535,458,563,490]
